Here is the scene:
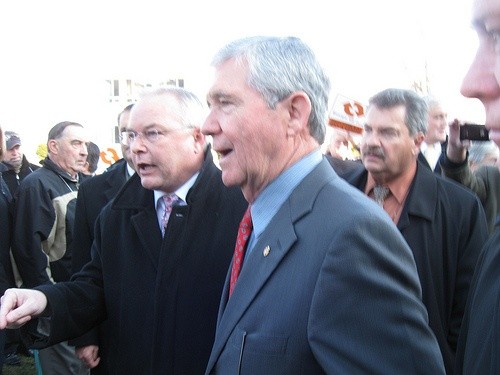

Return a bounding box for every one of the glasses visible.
[120,127,191,145]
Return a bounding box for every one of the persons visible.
[0,0,500,374]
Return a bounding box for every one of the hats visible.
[4,131,21,150]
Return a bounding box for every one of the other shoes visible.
[23,349,34,357]
[4,354,23,366]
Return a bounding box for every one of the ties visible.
[162,194,181,238]
[373,187,390,209]
[230,207,253,299]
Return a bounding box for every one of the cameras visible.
[459,124,489,141]
